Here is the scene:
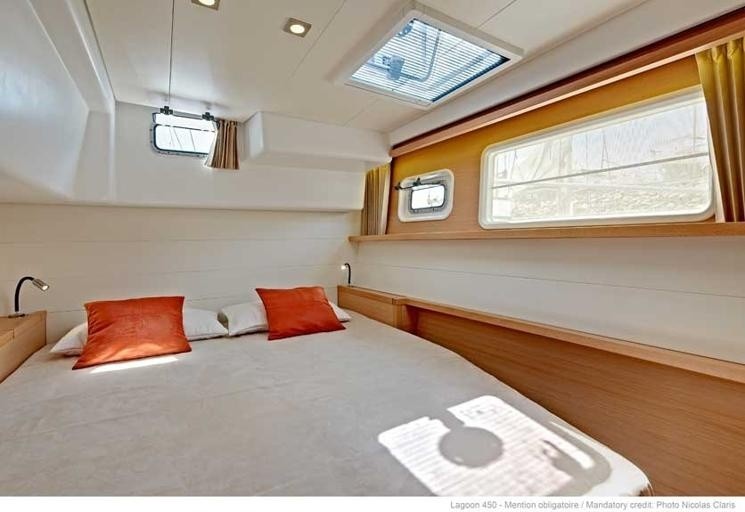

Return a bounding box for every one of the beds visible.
[0,301,661,497]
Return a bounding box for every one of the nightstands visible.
[1,312,46,383]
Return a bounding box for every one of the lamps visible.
[9,274,51,319]
